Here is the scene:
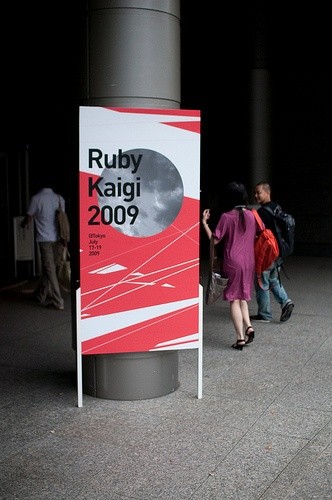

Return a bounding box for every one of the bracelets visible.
[204,224,208,228]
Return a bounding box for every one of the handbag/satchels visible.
[55,193,71,243]
[205,271,229,305]
[56,249,72,292]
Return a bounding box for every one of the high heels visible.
[232,338,245,351]
[243,328,254,345]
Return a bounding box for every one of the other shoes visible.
[281,302,295,321]
[249,314,271,323]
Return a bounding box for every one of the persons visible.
[203,184,265,350]
[20,179,65,311]
[249,182,295,322]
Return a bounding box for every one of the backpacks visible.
[265,206,295,258]
[251,210,279,289]
